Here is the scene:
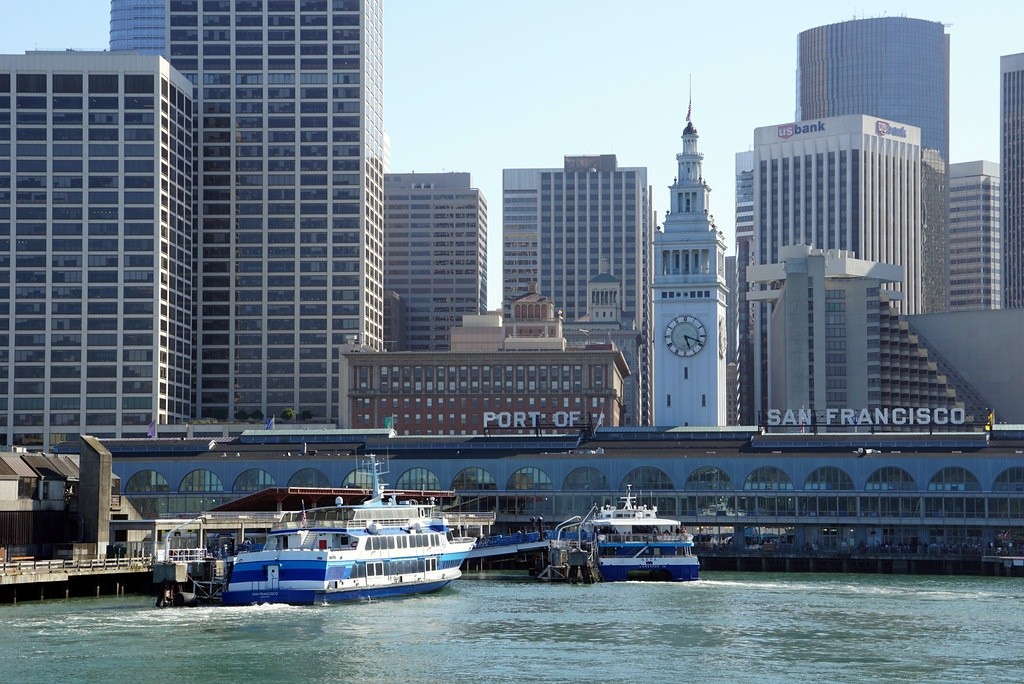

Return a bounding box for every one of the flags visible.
[265,418,275,430]
[147,419,157,438]
[385,416,394,429]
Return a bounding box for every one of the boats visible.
[223,454,478,606]
[589,484,700,583]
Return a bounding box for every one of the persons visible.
[697,541,731,552]
[476,535,486,548]
[859,539,1024,556]
[597,526,687,541]
[170,537,252,560]
[983,422,990,432]
[516,529,525,543]
[758,539,815,552]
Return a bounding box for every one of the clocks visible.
[666,316,705,357]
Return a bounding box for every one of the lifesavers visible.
[351,541,357,547]
